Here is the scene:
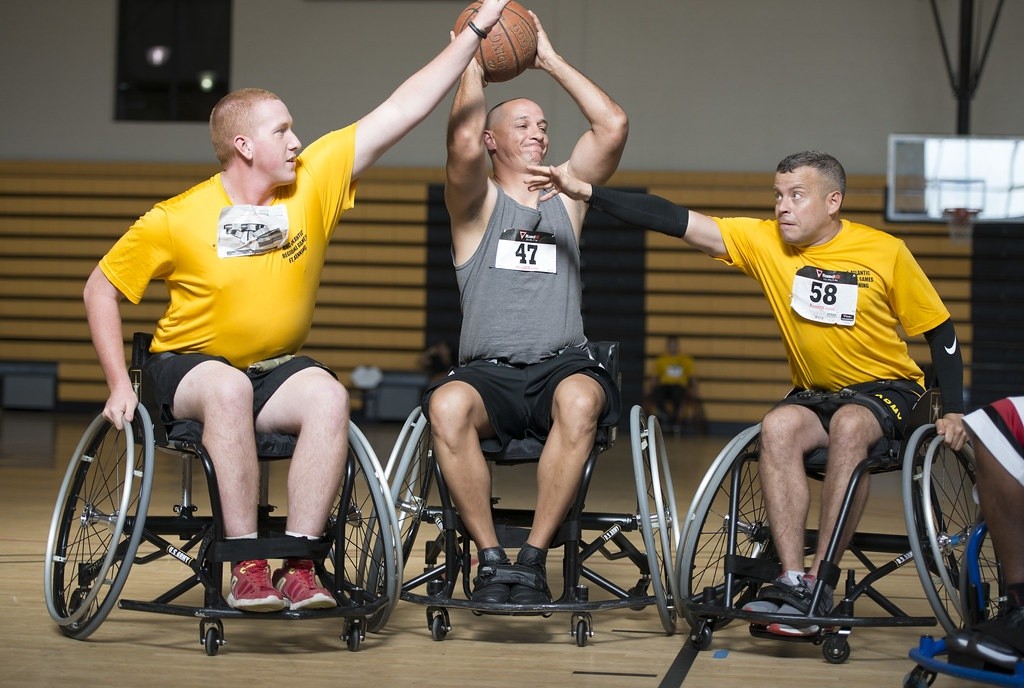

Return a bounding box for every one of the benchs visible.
[364,371,432,421]
[0,360,58,413]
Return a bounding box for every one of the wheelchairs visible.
[43,331,403,656]
[673,363,1007,664]
[354,341,681,647]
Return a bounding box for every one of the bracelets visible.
[468,21,487,39]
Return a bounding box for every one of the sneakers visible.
[740,570,834,639]
[955,609,1024,669]
[472,544,551,606]
[227,558,337,614]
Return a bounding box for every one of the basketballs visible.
[451,0,538,84]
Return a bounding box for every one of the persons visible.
[82,0,509,611]
[523,149,967,634]
[949,396,1024,665]
[422,10,629,606]
